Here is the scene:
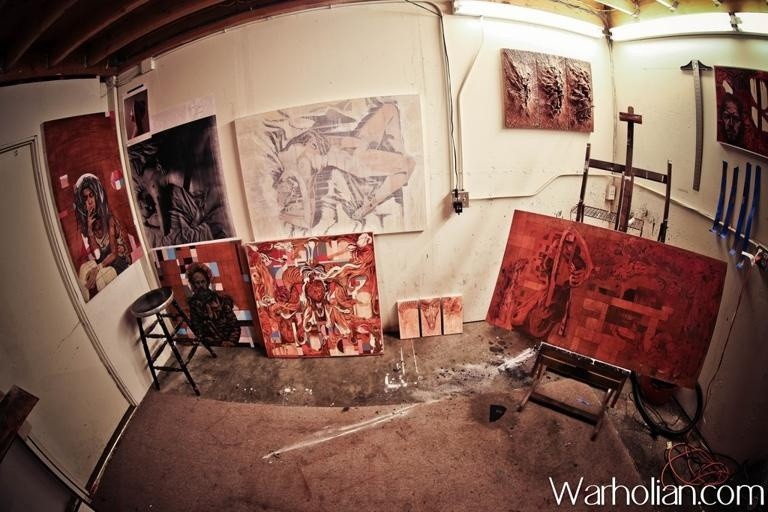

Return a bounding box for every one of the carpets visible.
[88,378,649,512]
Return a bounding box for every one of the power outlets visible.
[452,191,470,208]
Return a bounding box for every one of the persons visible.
[72,174,132,301]
[256,104,412,228]
[188,263,242,348]
[719,94,746,145]
[130,102,145,138]
[139,162,220,237]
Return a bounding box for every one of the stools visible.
[129,286,218,396]
